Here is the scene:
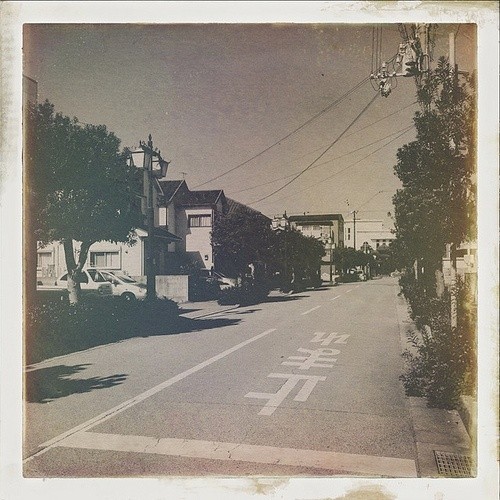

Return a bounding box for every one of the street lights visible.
[125,133,169,302]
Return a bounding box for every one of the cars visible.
[55,268,148,301]
